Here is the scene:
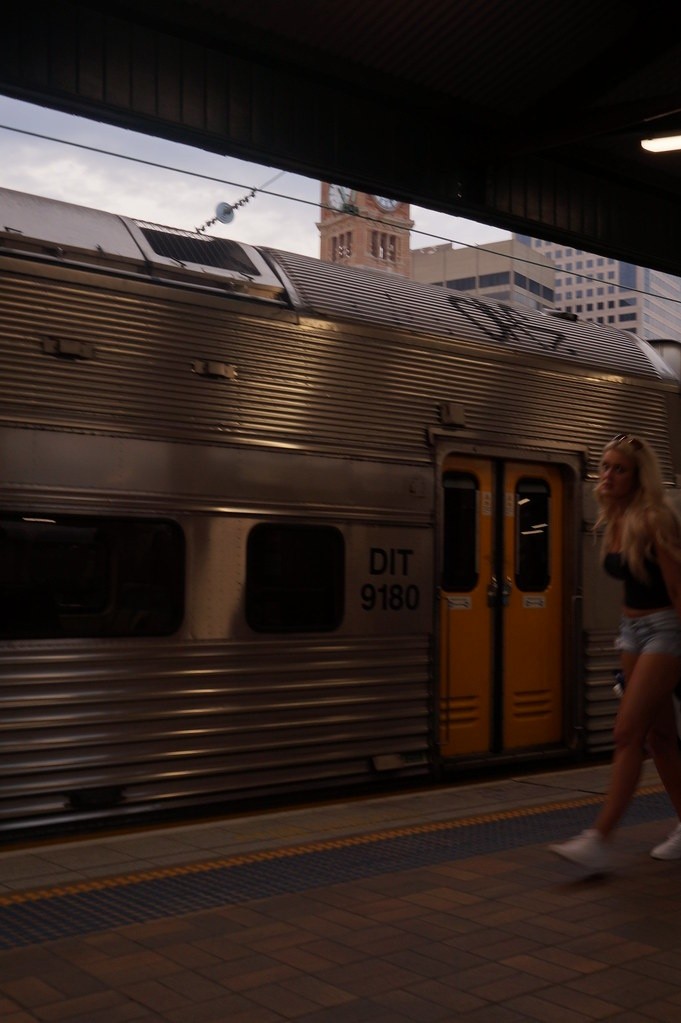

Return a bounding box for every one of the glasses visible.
[612,434,643,450]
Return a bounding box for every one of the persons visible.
[544,434,681,873]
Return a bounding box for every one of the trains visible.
[0,187,681,834]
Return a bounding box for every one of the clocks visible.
[327,185,356,214]
[373,195,402,213]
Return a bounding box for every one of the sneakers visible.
[549,829,619,873]
[651,823,681,859]
